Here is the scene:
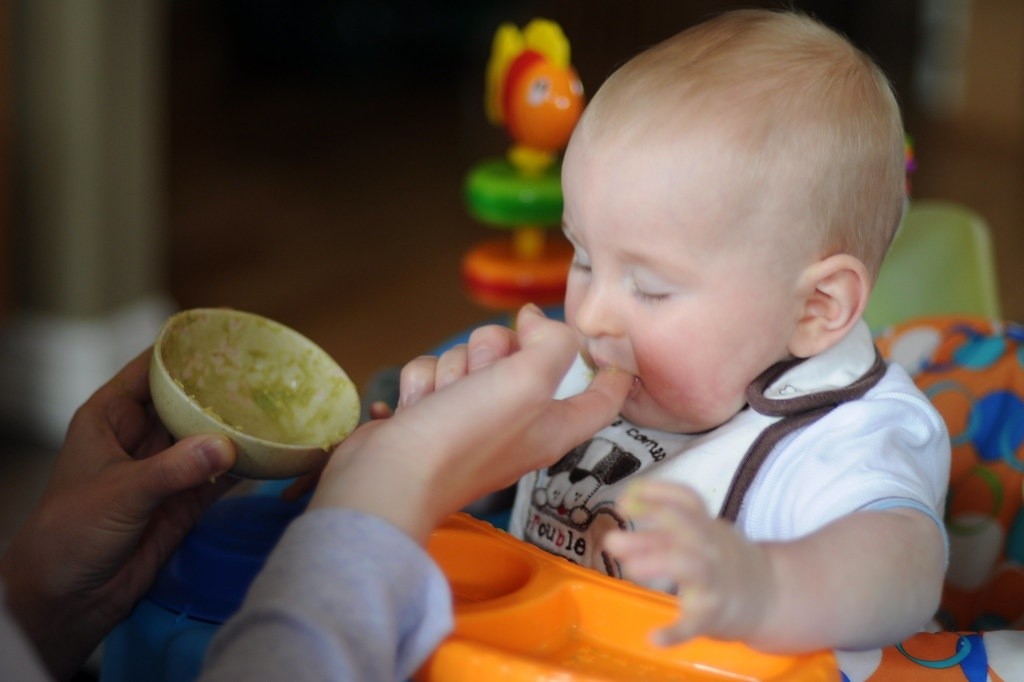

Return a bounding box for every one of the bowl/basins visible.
[149,307,361,481]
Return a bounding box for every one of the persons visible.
[280,9,950,655]
[0,303,633,682]
